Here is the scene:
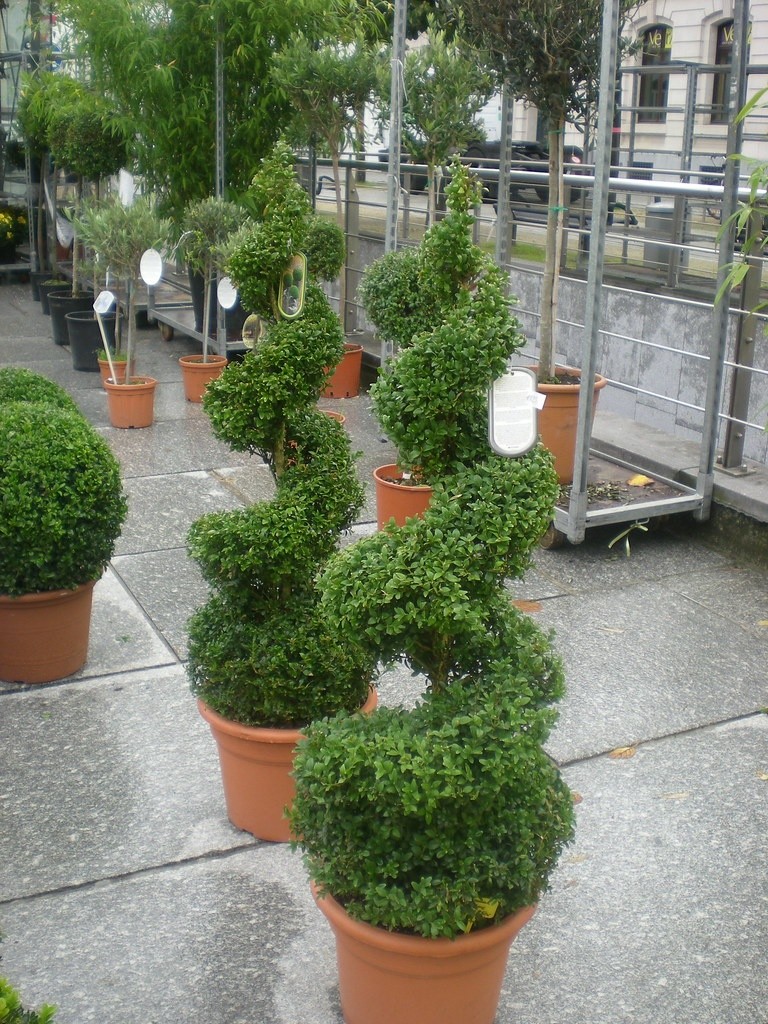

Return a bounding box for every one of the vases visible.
[0,239,15,261]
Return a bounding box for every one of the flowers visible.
[0,201,27,239]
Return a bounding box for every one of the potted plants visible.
[62,102,136,374]
[283,274,293,300]
[45,81,101,347]
[359,153,491,534]
[0,402,130,683]
[279,156,581,1024]
[86,193,168,429]
[438,0,649,489]
[92,197,135,392]
[17,71,80,301]
[68,0,386,342]
[30,74,77,314]
[183,146,377,845]
[287,286,299,314]
[309,217,365,400]
[178,199,243,403]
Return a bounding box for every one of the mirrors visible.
[490,365,538,456]
[279,251,308,319]
[242,314,262,348]
